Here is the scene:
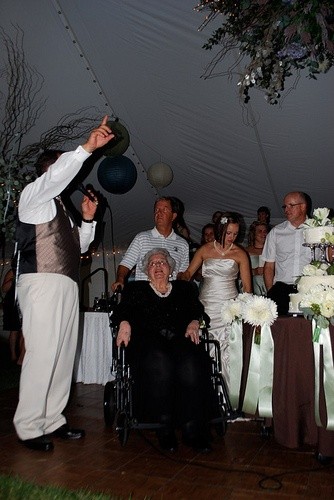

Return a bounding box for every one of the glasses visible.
[282,203,303,212]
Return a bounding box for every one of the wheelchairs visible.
[103,282,234,446]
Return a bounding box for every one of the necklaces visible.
[213,240,233,255]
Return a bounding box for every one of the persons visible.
[177,213,252,414]
[242,206,270,298]
[116,247,214,454]
[181,211,222,282]
[261,191,334,317]
[111,198,189,293]
[13,115,115,452]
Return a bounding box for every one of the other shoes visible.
[182,434,211,453]
[156,426,179,452]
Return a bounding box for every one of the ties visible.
[57,196,67,217]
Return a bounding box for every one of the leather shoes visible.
[49,424,85,439]
[19,436,53,452]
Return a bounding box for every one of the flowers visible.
[220,290,279,345]
[295,207,334,341]
[220,216,228,224]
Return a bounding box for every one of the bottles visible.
[93,296,99,311]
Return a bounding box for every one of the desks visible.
[72,311,118,385]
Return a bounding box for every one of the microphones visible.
[76,184,98,205]
